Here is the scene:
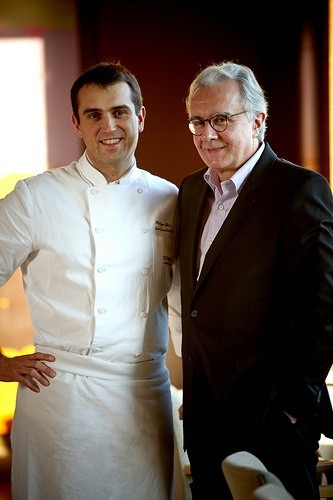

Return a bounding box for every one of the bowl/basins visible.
[319,440,333,458]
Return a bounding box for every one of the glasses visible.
[187,109,247,136]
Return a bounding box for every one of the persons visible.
[177,61,333,500]
[0,61,182,500]
